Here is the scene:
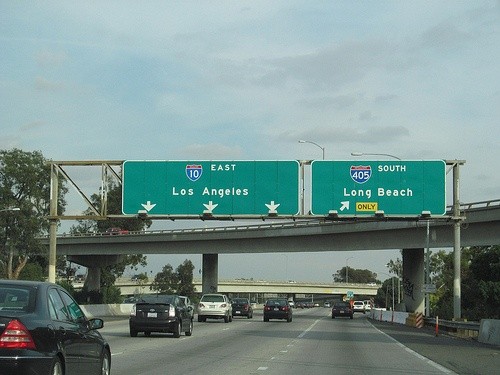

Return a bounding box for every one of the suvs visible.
[196,293,233,323]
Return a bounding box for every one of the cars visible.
[128,292,194,338]
[314,303,320,307]
[230,297,254,319]
[303,302,310,309]
[308,302,315,308]
[0,278,112,375]
[263,299,292,322]
[294,301,305,309]
[352,300,366,314]
[331,300,353,319]
[288,300,296,307]
[323,302,331,308]
[178,295,195,320]
[363,299,374,311]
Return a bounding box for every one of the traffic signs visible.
[309,159,447,218]
[120,159,301,217]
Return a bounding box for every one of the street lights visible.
[296,137,325,160]
